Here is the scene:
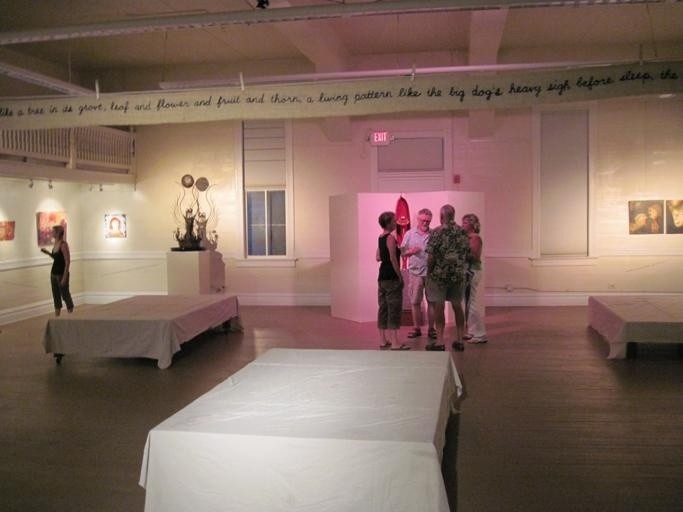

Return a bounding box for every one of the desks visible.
[586,292,682,362]
[42,294,241,371]
[139,346,465,512]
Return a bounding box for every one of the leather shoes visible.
[451,340,464,352]
[425,342,445,351]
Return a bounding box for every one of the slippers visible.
[391,344,412,350]
[380,341,392,348]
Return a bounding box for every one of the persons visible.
[425,205,472,351]
[461,214,488,344]
[105,217,124,238]
[41,226,74,316]
[376,212,411,350]
[400,209,437,339]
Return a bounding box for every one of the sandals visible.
[407,327,422,339]
[427,327,437,340]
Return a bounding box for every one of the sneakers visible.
[462,332,488,344]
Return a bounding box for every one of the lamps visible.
[47,178,52,191]
[28,178,34,188]
